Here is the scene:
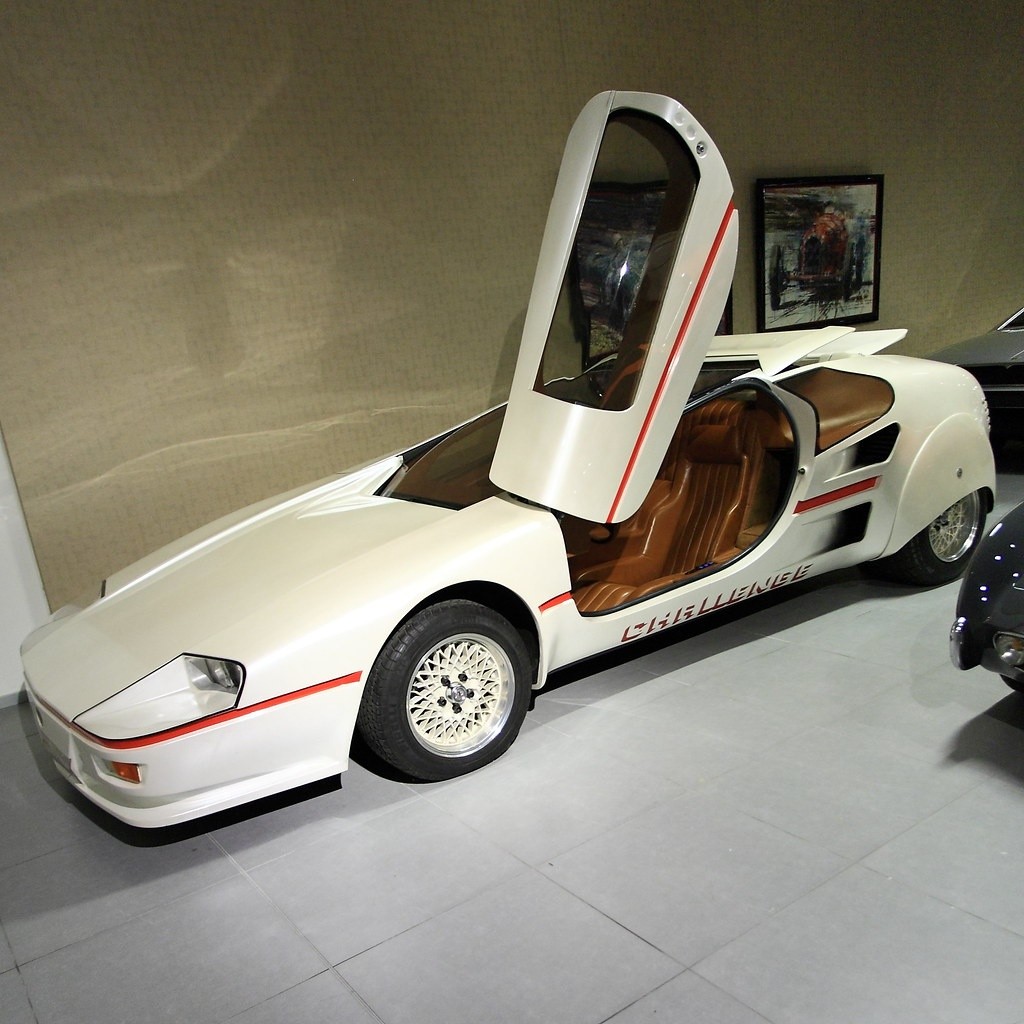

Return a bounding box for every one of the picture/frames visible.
[756,173,884,333]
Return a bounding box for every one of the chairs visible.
[574,399,771,609]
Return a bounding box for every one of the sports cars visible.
[14,86,998,833]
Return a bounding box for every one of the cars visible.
[948,500,1024,700]
[918,306,1023,449]
